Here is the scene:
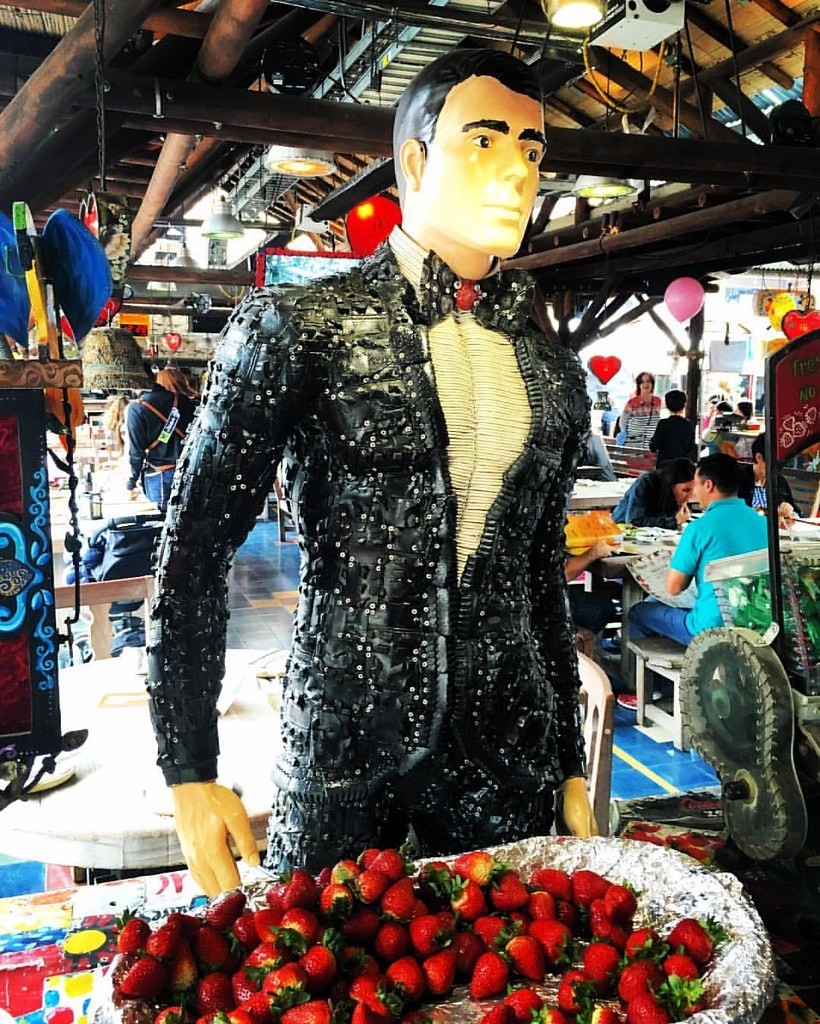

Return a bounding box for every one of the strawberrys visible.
[112,846,731,1024]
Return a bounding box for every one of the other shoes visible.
[653,692,667,701]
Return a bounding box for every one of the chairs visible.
[627,636,692,752]
[575,650,616,838]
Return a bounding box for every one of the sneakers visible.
[617,693,636,710]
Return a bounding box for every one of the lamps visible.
[146,254,177,292]
[541,0,604,29]
[261,145,339,176]
[173,199,200,267]
[200,193,245,239]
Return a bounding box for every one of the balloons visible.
[782,310,820,340]
[665,277,705,321]
[588,355,621,385]
[346,195,402,256]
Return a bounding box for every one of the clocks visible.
[124,284,134,299]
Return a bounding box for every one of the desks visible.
[0,860,276,1024]
[567,478,643,508]
[0,649,295,868]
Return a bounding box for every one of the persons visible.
[619,372,661,449]
[564,542,620,636]
[125,383,196,511]
[616,452,752,710]
[144,48,598,897]
[157,369,197,399]
[611,458,696,530]
[578,435,617,481]
[738,433,801,519]
[648,390,694,466]
[701,395,752,456]
